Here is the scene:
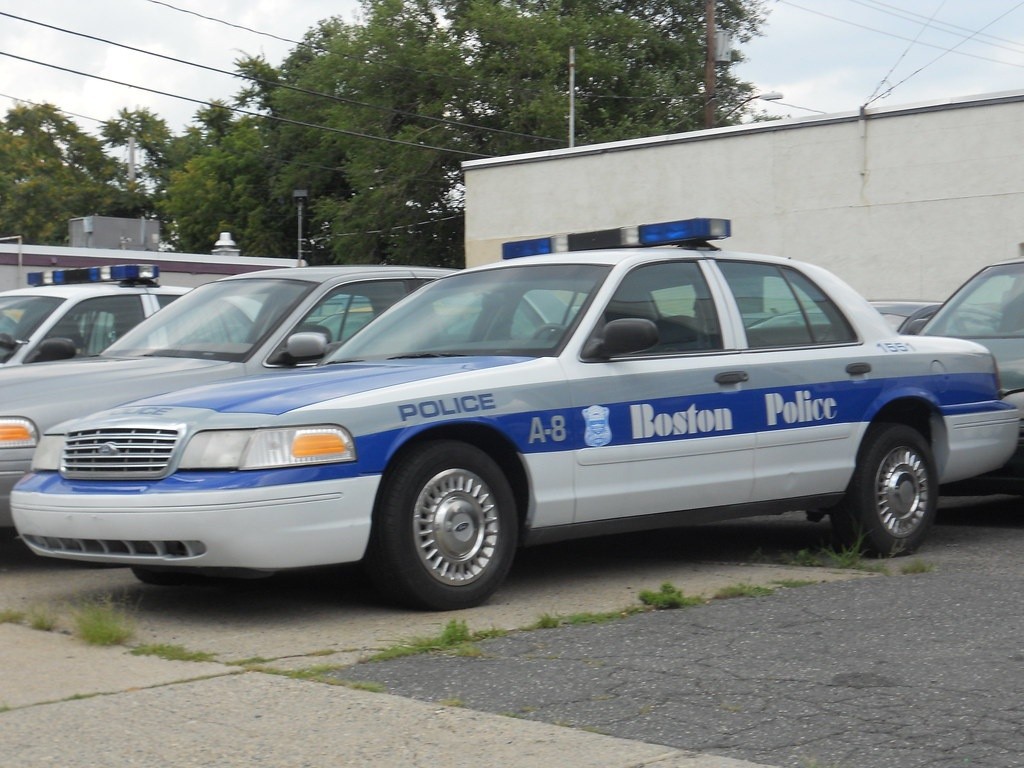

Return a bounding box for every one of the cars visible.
[739,258,1022,422]
[0,267,577,532]
[10,215,1020,613]
